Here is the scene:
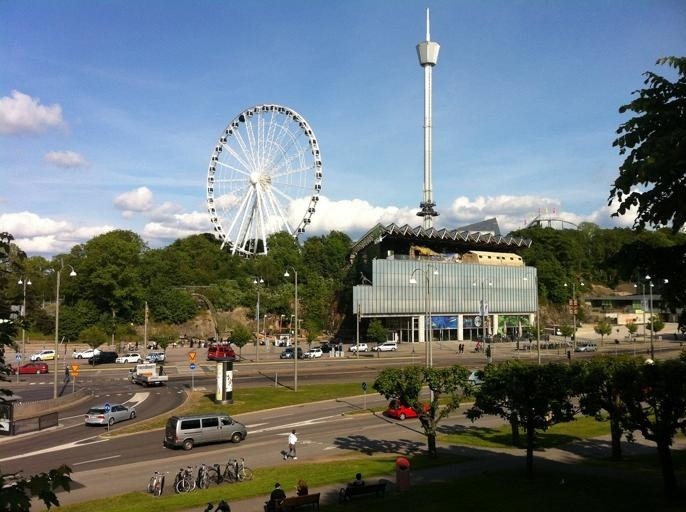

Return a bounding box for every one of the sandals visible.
[557,328,563,336]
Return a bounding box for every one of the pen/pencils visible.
[207,344,236,361]
[163,412,247,451]
[575,342,598,352]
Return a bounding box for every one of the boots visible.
[387,399,434,419]
[303,349,324,358]
[88,352,117,364]
[145,352,167,363]
[84,403,136,425]
[371,342,399,352]
[115,353,142,364]
[30,349,59,361]
[280,347,302,359]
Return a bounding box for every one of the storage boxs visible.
[264,493,321,512]
[339,482,387,505]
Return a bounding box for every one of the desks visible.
[279,315,286,333]
[409,265,439,368]
[290,314,295,334]
[254,276,265,362]
[522,272,541,365]
[263,314,267,332]
[53,264,77,399]
[634,282,651,343]
[283,265,297,391]
[473,278,493,354]
[17,274,32,354]
[646,275,670,360]
[299,319,304,329]
[563,281,585,350]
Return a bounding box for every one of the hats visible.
[73,349,100,359]
[12,362,48,374]
[474,333,520,342]
[465,368,490,386]
[349,342,368,352]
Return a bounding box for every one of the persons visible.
[459,344,462,353]
[476,341,481,352]
[283,430,298,460]
[267,483,285,512]
[348,474,365,488]
[63,365,70,382]
[296,480,308,496]
[462,345,464,354]
